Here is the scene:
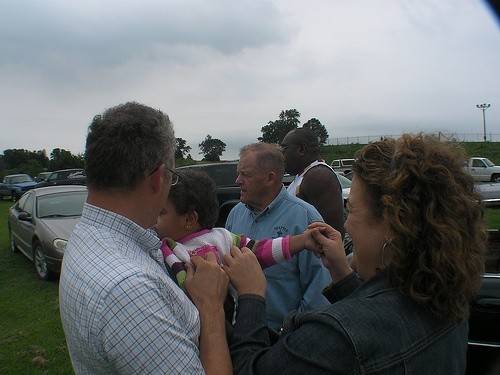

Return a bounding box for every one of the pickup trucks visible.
[466,157,500,183]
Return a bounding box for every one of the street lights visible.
[476,103,491,142]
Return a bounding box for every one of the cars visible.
[38,169,87,189]
[34,172,53,183]
[466,229,500,352]
[8,185,88,282]
[173,159,358,230]
[0,174,39,202]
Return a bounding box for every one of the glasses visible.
[148,166,180,186]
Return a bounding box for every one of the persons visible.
[153,169,326,304]
[281,128,347,241]
[223,141,324,343]
[220,131,485,375]
[58,102,234,375]
